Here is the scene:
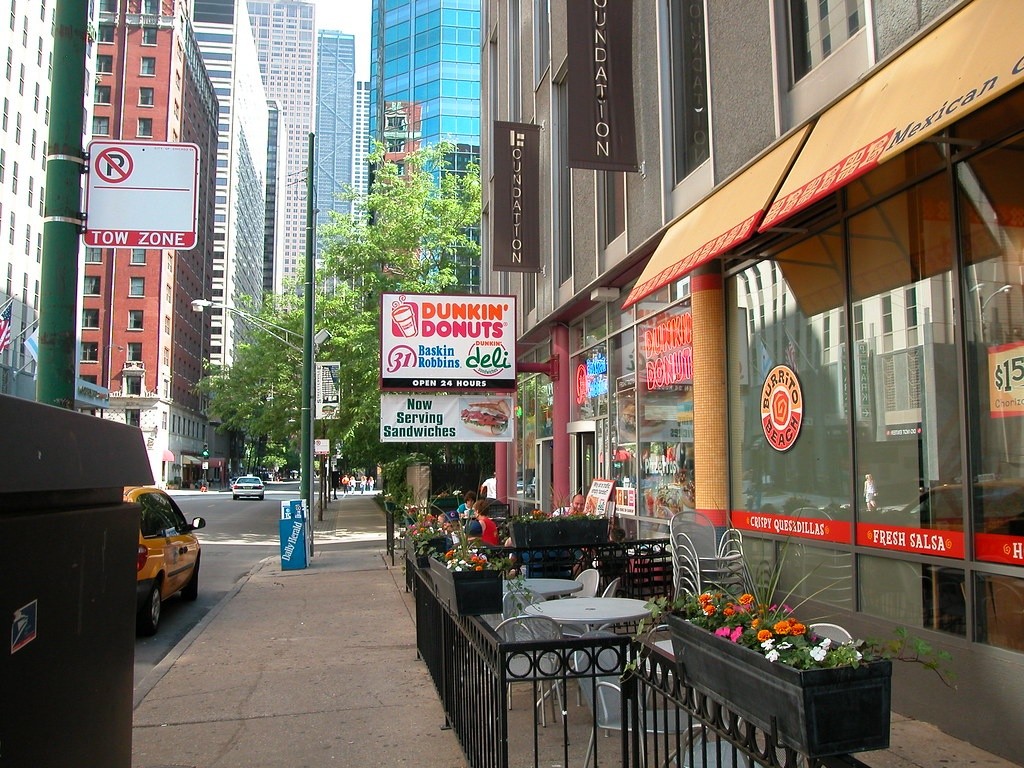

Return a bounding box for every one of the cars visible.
[232,474,265,500]
[900,477,1024,537]
[123,485,206,639]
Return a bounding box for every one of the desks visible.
[503,578,583,601]
[524,596,653,725]
[625,640,750,768]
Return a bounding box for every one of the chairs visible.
[495,510,855,768]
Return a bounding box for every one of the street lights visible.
[192,298,317,557]
[203,443,210,458]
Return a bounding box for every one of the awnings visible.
[162,450,201,465]
[621,0,1023,312]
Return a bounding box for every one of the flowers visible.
[617,488,959,694]
[397,487,606,627]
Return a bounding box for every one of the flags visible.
[0,300,12,355]
[23,325,40,381]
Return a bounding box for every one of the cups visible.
[450,531,465,546]
[629,491,634,505]
[624,490,628,505]
[618,490,622,505]
[623,478,630,487]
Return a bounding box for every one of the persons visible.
[360,476,374,494]
[863,474,878,511]
[342,474,356,494]
[480,472,496,501]
[613,466,764,527]
[437,488,626,579]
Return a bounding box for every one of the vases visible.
[666,613,893,761]
[429,497,464,508]
[428,555,503,618]
[405,537,446,569]
[402,513,416,527]
[509,519,610,547]
